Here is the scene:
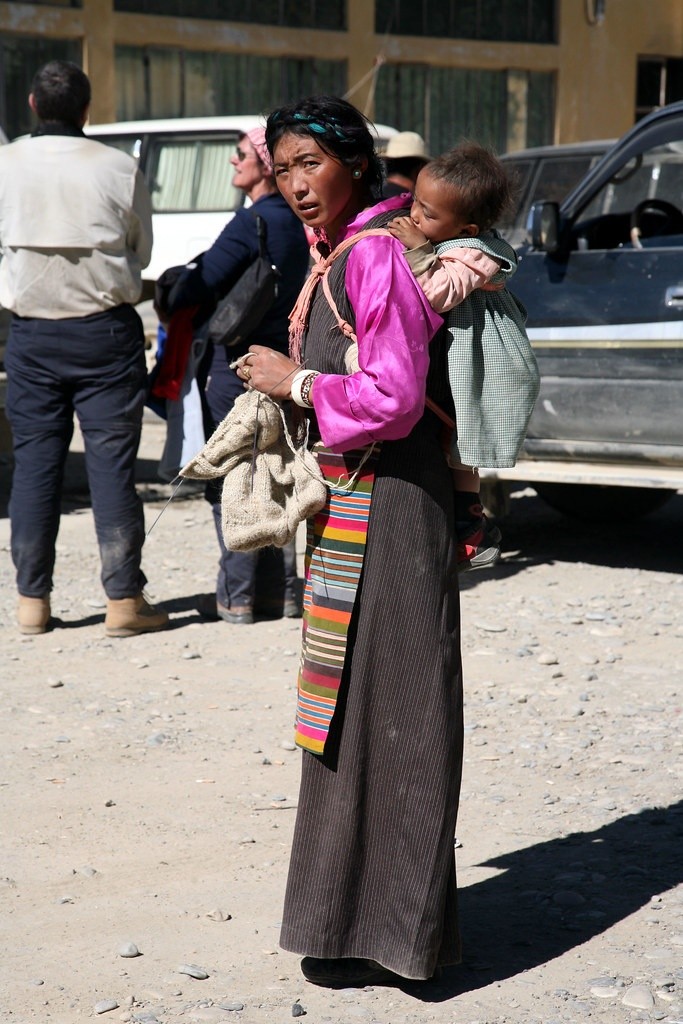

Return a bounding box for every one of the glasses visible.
[236,148,255,161]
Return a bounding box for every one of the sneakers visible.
[104,589,169,637]
[254,592,300,617]
[16,592,51,635]
[195,594,253,623]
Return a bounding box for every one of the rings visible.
[242,367,251,376]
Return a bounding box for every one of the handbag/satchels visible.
[207,209,282,347]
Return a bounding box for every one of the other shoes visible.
[302,956,448,995]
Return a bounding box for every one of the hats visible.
[377,132,434,163]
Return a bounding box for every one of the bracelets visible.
[290,370,321,408]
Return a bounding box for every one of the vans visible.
[477,100,683,513]
[13,117,399,512]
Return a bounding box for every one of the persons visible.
[154,98,467,981]
[387,142,541,562]
[1,60,169,638]
